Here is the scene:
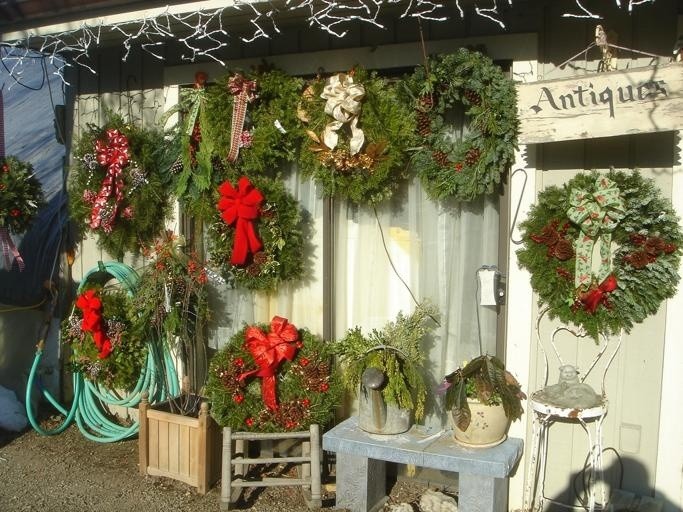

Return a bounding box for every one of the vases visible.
[449,395,509,449]
[139,377,235,496]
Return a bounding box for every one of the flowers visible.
[298,66,514,202]
[200,321,339,434]
[62,69,301,393]
[515,172,681,345]
[0,156,47,235]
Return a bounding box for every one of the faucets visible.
[43,280,59,304]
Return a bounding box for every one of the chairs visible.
[524,306,625,510]
